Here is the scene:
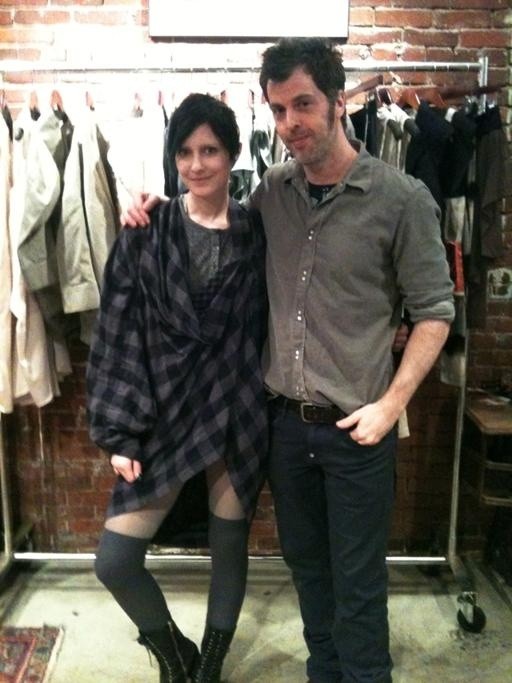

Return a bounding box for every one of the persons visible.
[83,92,270,683]
[121,38,456,683]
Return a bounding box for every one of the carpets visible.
[0,625,63,683]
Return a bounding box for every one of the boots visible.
[136,620,237,683]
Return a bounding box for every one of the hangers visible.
[0,63,480,148]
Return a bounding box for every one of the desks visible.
[464,393,512,588]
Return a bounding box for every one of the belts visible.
[271,395,345,424]
